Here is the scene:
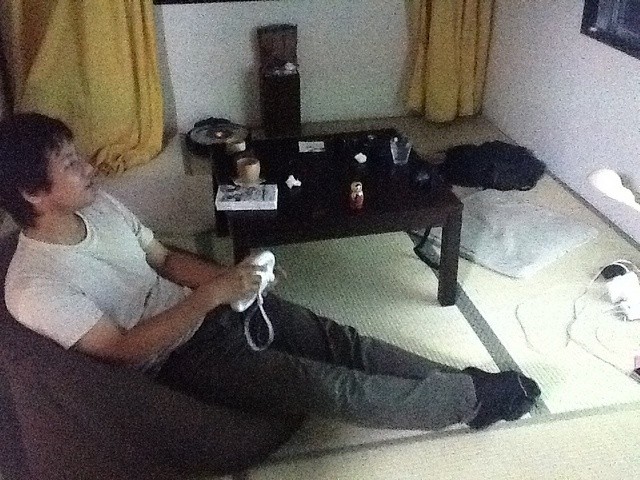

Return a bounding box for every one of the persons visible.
[1,113,542,430]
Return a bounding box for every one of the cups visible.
[390,136,413,164]
[236,158,261,184]
[224,136,246,156]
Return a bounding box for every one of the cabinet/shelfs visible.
[261,69,302,136]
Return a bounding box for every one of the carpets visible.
[408,190,596,278]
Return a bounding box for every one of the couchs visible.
[0,230,309,480]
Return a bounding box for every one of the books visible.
[215,184,278,211]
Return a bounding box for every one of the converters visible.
[605,272,640,304]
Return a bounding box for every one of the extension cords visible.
[600,260,640,322]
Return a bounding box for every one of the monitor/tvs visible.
[580,1,636,58]
